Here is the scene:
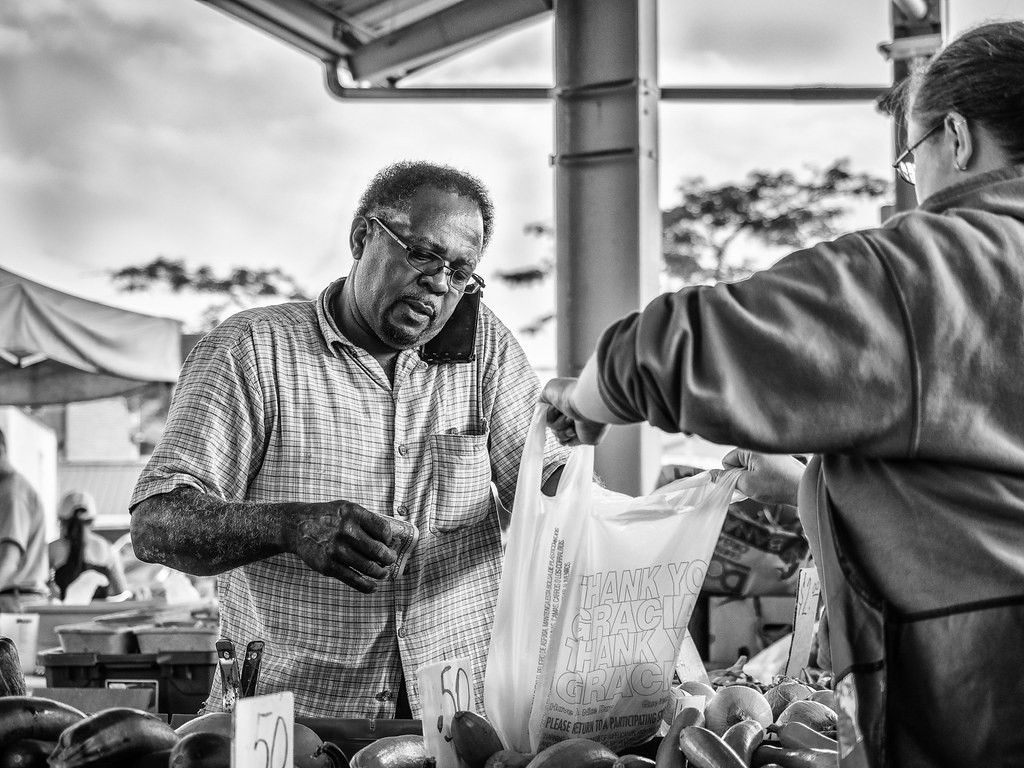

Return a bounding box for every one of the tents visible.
[0,266,185,412]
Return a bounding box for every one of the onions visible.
[664,673,839,738]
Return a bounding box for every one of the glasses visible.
[894,117,949,188]
[365,215,484,294]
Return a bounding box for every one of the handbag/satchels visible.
[480,403,750,759]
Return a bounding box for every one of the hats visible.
[58,491,96,523]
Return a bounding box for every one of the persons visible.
[125,160,600,730]
[0,430,53,615]
[42,490,124,603]
[535,16,1024,768]
